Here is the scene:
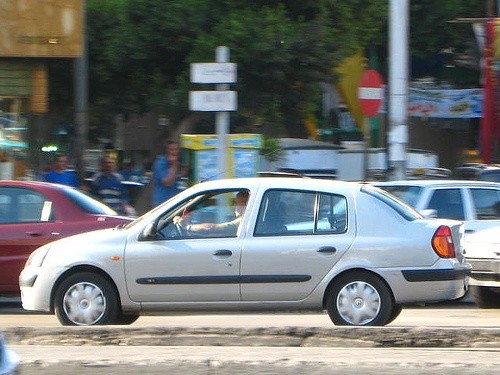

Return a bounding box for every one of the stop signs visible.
[356,68,384,117]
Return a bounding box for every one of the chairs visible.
[260,197,286,233]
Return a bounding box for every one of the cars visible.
[18,177,473,326]
[354,181,500,290]
[0,178,138,299]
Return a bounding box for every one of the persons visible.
[28,143,118,194]
[79,154,139,216]
[151,140,192,210]
[172,190,250,234]
